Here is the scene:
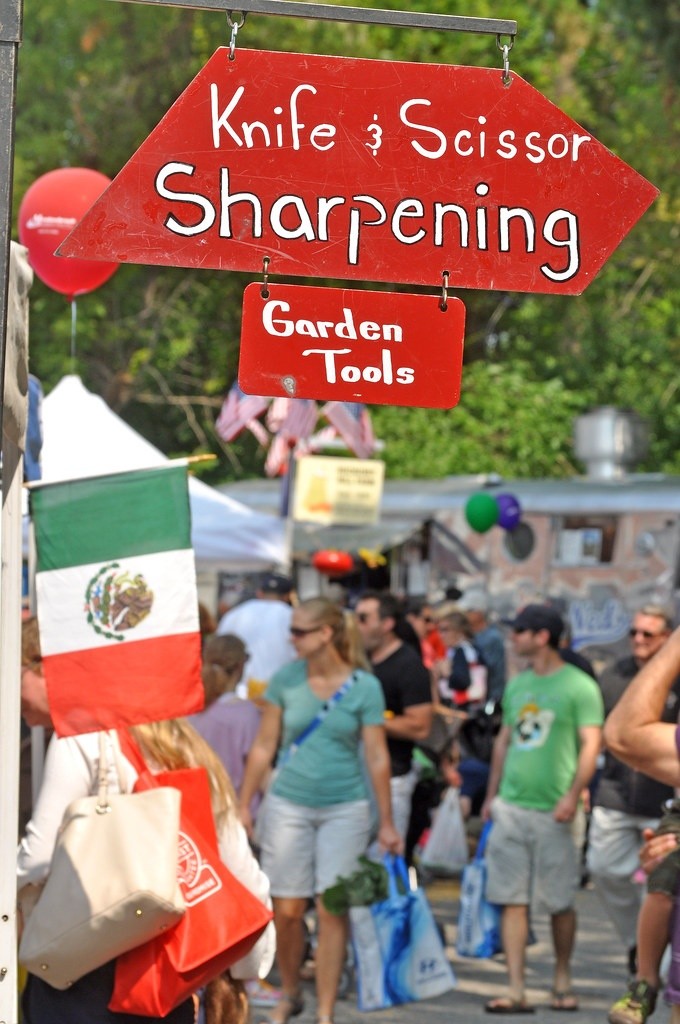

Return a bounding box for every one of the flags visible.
[29,463,208,740]
[214,377,375,477]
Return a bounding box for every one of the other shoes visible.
[625,945,641,974]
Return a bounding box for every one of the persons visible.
[186,576,507,1024]
[16,619,277,1024]
[478,603,680,1024]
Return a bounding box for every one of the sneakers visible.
[607,981,661,1024]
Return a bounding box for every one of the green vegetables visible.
[322,858,390,916]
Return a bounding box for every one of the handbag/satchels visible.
[15,727,278,1019]
[457,823,537,957]
[347,857,459,1010]
[437,643,489,705]
[421,787,470,878]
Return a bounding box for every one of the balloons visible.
[465,490,535,562]
[20,166,122,298]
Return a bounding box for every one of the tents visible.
[292,515,495,584]
[21,371,296,577]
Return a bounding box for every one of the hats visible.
[501,605,562,636]
[261,574,292,593]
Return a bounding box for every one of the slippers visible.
[552,985,577,1012]
[484,994,537,1013]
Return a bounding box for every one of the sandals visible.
[260,993,307,1024]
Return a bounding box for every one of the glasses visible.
[514,625,539,635]
[358,611,381,624]
[630,628,666,639]
[289,626,323,639]
